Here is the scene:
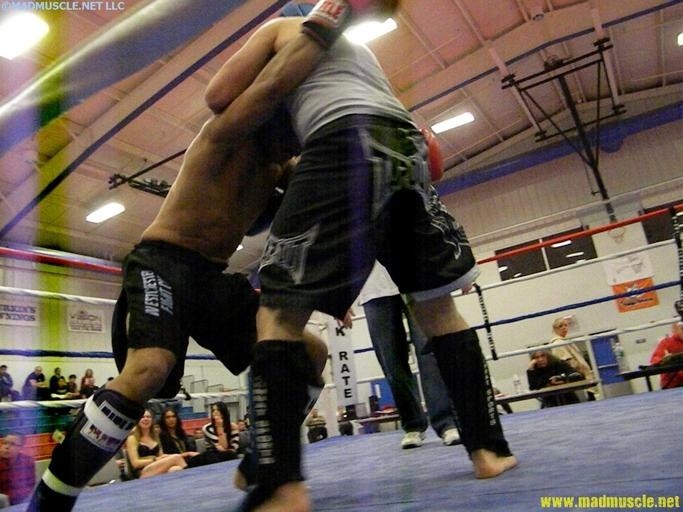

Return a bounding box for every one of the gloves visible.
[416,128,445,182]
[300,0,381,49]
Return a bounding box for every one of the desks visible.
[356,377,598,429]
[615,360,683,392]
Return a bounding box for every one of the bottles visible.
[615,342,627,373]
[512,374,521,394]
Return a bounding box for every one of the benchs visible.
[0,397,213,459]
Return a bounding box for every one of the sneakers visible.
[441,427,461,446]
[400,431,428,448]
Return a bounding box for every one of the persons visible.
[0,363,251,512]
[24,1,402,512]
[650,322,683,389]
[336,406,353,435]
[336,258,472,449]
[526,345,586,409]
[304,410,327,443]
[551,319,600,401]
[205,16,518,512]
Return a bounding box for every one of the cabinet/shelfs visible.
[528,328,634,401]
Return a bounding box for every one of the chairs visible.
[0,438,206,512]
